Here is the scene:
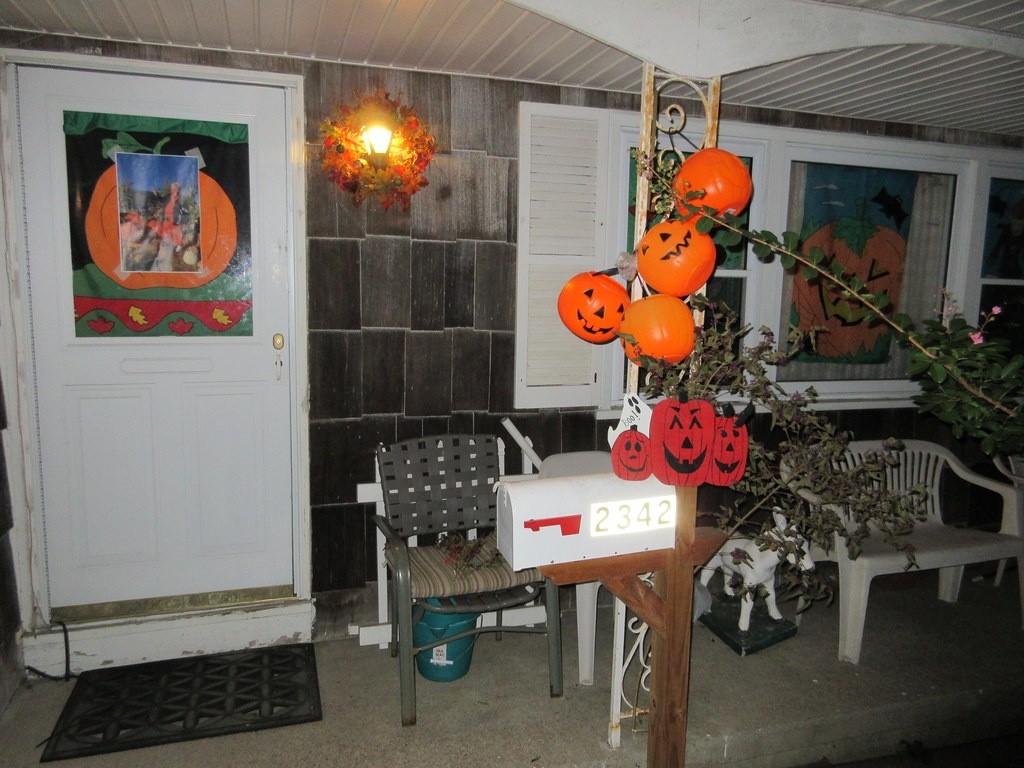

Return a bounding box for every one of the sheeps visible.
[698,506,816,637]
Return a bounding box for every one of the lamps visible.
[357,105,399,171]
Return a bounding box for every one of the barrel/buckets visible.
[412,598,483,683]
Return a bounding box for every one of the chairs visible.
[372,430,568,722]
[782,434,1024,670]
